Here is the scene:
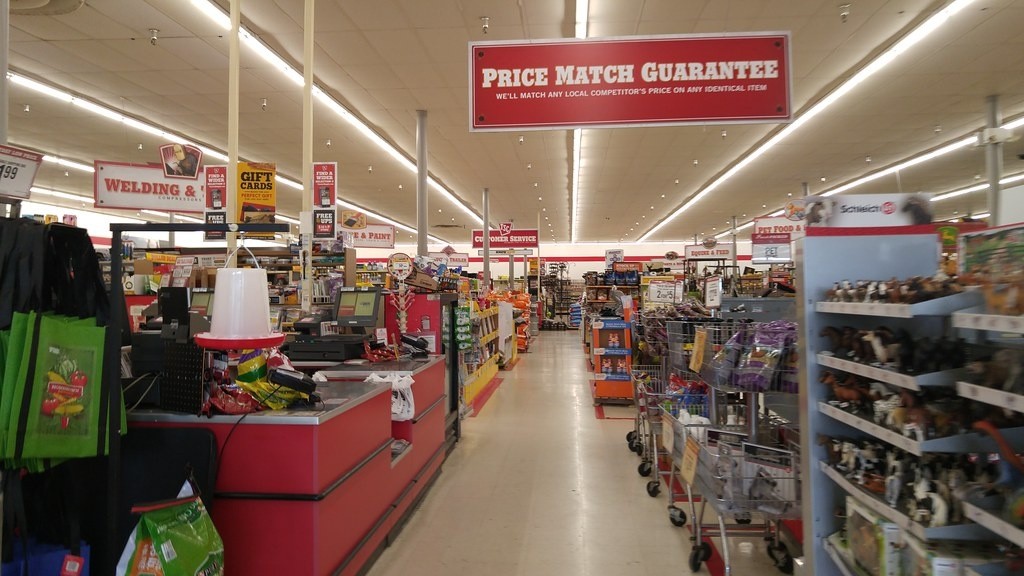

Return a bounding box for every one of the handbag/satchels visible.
[0,217,127,472]
[0,467,90,576]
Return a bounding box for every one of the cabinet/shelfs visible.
[95,221,1024,576]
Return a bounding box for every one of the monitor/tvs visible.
[189,288,216,323]
[333,286,382,334]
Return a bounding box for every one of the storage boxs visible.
[962,558,1024,576]
[898,526,961,576]
[122,274,154,295]
[742,441,799,515]
[705,427,750,487]
[844,494,903,576]
[942,538,987,567]
[973,541,1009,565]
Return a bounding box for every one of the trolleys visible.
[626,365,801,574]
